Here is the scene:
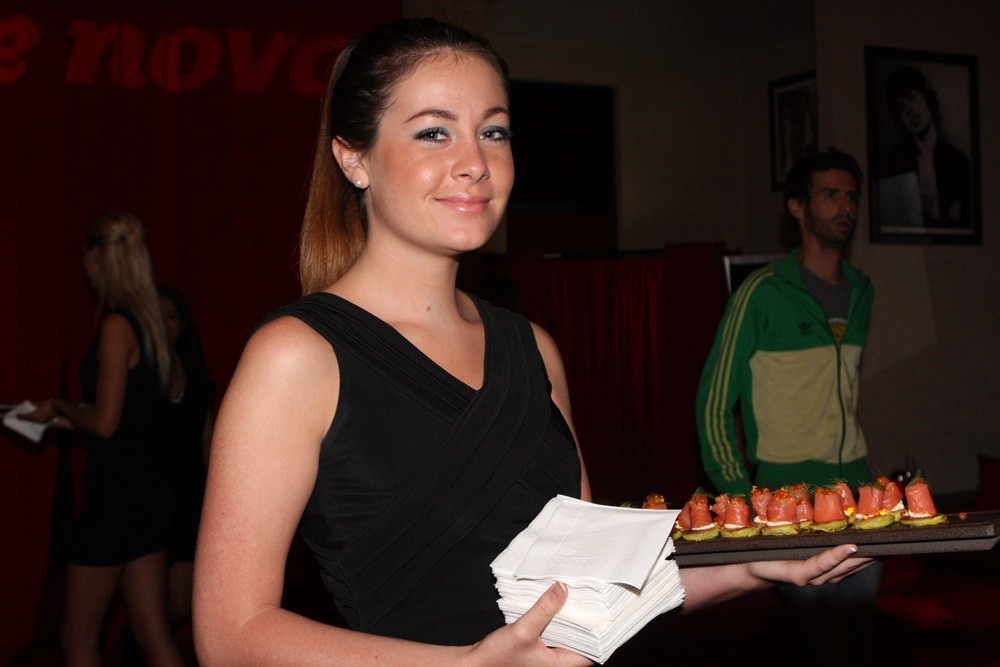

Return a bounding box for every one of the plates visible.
[668,510,1000,568]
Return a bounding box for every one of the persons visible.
[192,17,877,667]
[15,214,215,667]
[699,148,884,667]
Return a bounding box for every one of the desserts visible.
[644,470,949,540]
